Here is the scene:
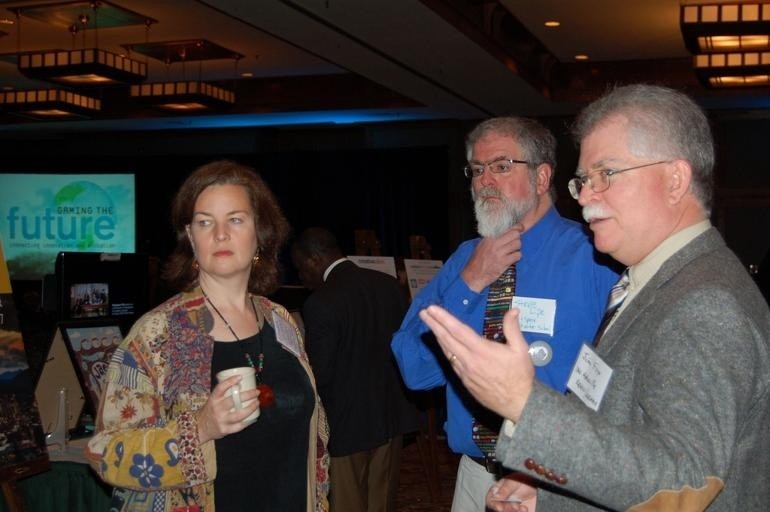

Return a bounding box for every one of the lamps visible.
[679,0,770,55]
[0,49,102,121]
[120,38,246,113]
[6,0,159,87]
[692,52,770,87]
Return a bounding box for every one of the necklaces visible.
[200,289,276,409]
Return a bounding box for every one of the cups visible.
[216,367,261,424]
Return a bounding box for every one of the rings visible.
[449,355,458,364]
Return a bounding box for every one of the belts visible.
[468,456,500,476]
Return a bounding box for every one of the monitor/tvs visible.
[46,253,150,322]
[0,166,143,281]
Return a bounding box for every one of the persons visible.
[290,225,418,512]
[419,83,770,512]
[393,114,619,512]
[88,159,330,512]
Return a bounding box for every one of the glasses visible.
[463,153,536,179]
[568,158,672,200]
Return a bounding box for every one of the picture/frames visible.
[58,321,128,420]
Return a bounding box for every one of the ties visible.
[589,267,630,350]
[470,262,517,462]
